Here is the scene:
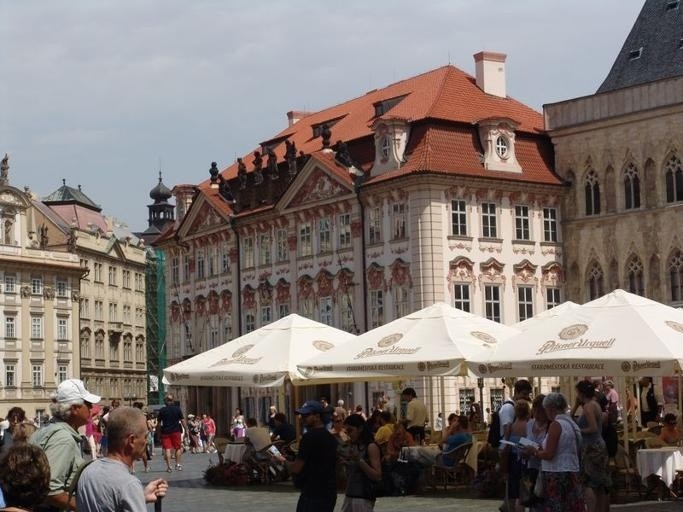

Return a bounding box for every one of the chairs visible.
[618,426,642,462]
[289,439,298,459]
[475,441,496,474]
[434,442,473,490]
[397,445,423,493]
[215,437,231,466]
[645,425,683,448]
[250,440,286,486]
[614,443,651,495]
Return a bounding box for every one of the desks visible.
[225,441,248,466]
[471,430,489,441]
[636,446,683,501]
[400,441,484,479]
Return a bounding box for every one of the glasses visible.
[665,421,678,426]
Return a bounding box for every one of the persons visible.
[0,406,26,443]
[265,405,277,433]
[186,414,201,454]
[496,380,532,512]
[0,443,50,512]
[336,413,381,511]
[75,407,169,512]
[414,416,472,498]
[657,412,683,447]
[397,387,430,446]
[101,399,121,424]
[272,399,341,512]
[271,412,296,447]
[569,380,606,510]
[505,400,532,512]
[333,400,347,433]
[187,414,199,454]
[521,392,583,512]
[242,418,273,485]
[129,448,154,475]
[320,397,338,433]
[599,380,618,468]
[26,377,98,509]
[231,407,247,442]
[156,393,189,473]
[441,413,460,444]
[592,379,608,412]
[383,418,414,498]
[202,412,218,454]
[373,410,395,458]
[352,404,367,421]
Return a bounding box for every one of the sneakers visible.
[174,464,179,470]
[131,472,135,476]
[144,468,151,473]
[179,465,184,472]
[166,467,173,472]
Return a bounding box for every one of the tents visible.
[295,300,523,450]
[463,287,683,493]
[161,312,358,441]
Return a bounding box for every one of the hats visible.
[188,414,195,419]
[294,400,324,415]
[53,379,102,405]
